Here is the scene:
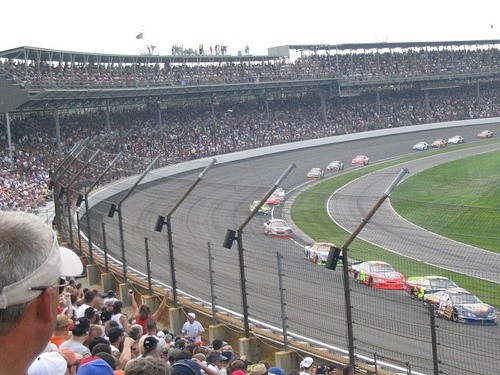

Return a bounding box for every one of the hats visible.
[230,370,244,375]
[144,337,159,347]
[67,353,83,365]
[171,359,201,375]
[301,357,314,368]
[80,356,100,366]
[101,311,111,319]
[250,364,267,375]
[74,317,90,333]
[157,331,164,336]
[76,359,115,375]
[46,343,58,352]
[268,367,285,375]
[0,236,83,309]
[188,313,196,319]
[56,315,72,328]
[208,354,228,362]
[94,351,116,370]
[27,352,67,375]
[59,349,74,360]
[231,360,244,370]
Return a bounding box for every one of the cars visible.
[303,242,343,267]
[327,160,344,171]
[350,260,405,290]
[431,139,448,148]
[403,276,460,301]
[307,168,325,179]
[265,188,285,207]
[263,218,293,238]
[422,288,498,326]
[413,141,429,151]
[249,201,271,217]
[477,130,493,139]
[352,155,369,166]
[448,136,464,144]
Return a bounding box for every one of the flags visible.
[136,33,143,39]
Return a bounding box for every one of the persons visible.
[0,43,500,213]
[28,276,380,375]
[1,211,85,375]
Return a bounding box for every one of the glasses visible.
[29,277,69,293]
[119,334,124,337]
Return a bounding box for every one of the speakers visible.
[59,187,65,198]
[324,247,341,270]
[108,203,116,218]
[223,229,236,249]
[49,180,54,190]
[76,194,83,207]
[49,170,52,177]
